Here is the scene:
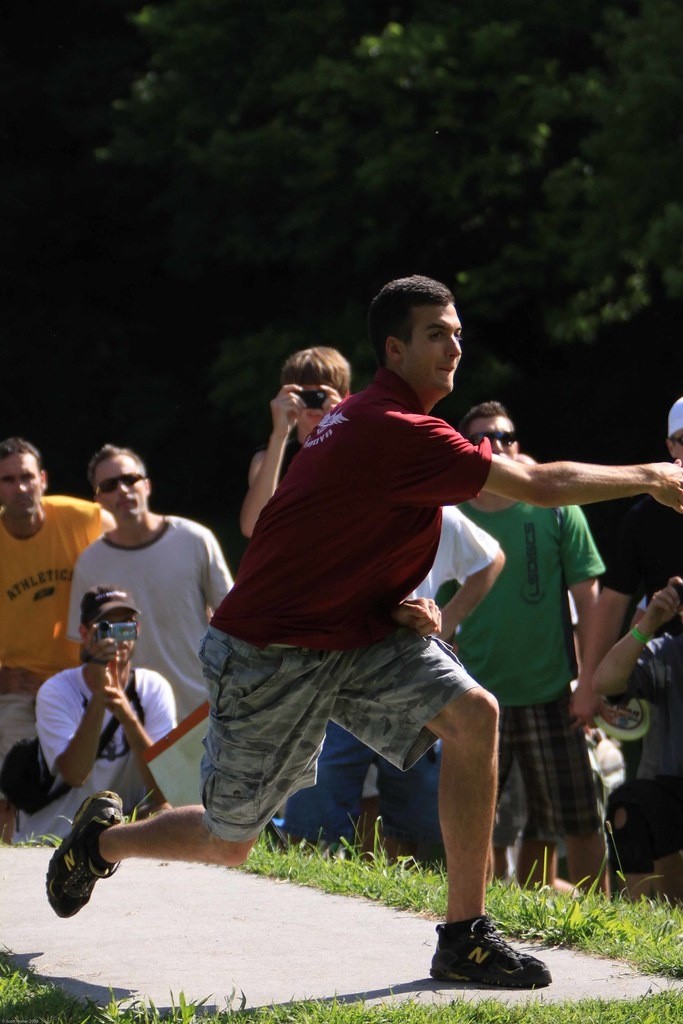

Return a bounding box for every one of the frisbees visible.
[593,693,651,742]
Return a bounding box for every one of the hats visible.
[79,585,140,627]
[667,397,683,436]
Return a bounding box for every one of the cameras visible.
[95,621,137,643]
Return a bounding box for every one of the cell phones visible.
[298,390,327,409]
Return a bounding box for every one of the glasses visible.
[669,436,683,445]
[96,473,144,496]
[467,431,517,450]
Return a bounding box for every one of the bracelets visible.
[632,624,653,643]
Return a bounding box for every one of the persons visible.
[0,339,683,897]
[48,275,683,988]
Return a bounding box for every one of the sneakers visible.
[430,917,552,987]
[46,791,123,918]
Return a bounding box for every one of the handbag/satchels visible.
[0,738,53,815]
